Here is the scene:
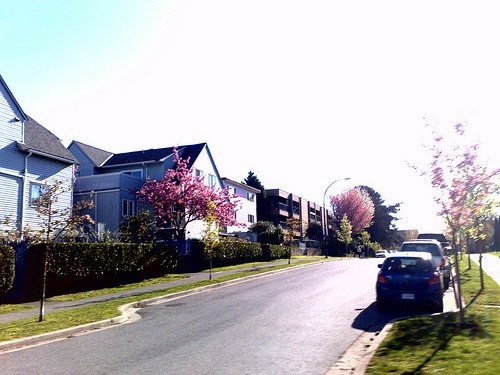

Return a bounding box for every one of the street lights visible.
[323,177,352,259]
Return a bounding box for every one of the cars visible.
[375,250,448,313]
[376,249,398,258]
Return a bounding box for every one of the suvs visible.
[399,237,452,290]
[416,232,451,260]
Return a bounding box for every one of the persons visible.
[353,244,362,259]
[439,258,449,291]
